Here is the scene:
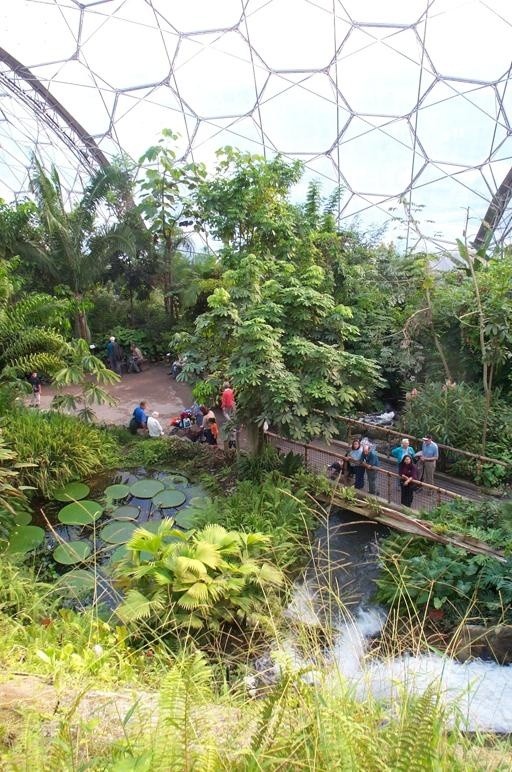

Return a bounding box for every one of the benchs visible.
[116,358,149,376]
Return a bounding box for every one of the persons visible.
[345,440,380,496]
[390,434,439,506]
[166,351,188,380]
[106,336,143,377]
[130,400,219,446]
[29,370,42,409]
[221,382,237,424]
